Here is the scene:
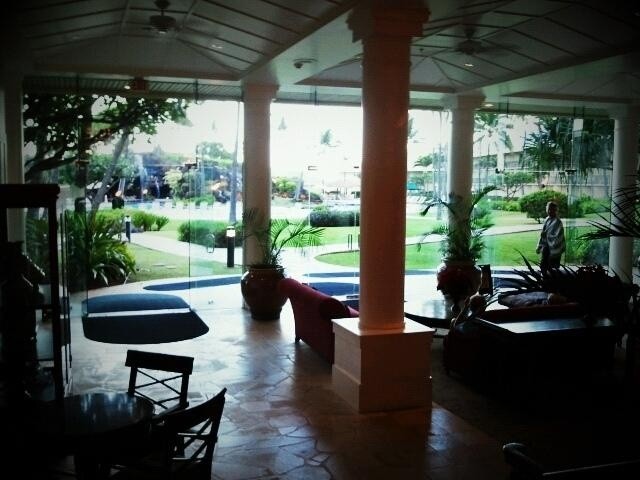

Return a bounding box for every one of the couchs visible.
[495,267,640,312]
[279,275,364,366]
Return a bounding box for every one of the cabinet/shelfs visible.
[0,181,74,401]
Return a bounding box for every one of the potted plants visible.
[221,205,326,325]
[419,184,510,301]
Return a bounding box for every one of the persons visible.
[112,190,125,211]
[450,296,488,333]
[536,201,567,274]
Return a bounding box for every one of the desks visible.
[0,391,152,480]
[467,300,625,396]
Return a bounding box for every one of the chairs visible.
[133,380,226,478]
[99,347,195,477]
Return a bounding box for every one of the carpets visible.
[306,281,360,313]
[419,324,639,440]
[78,292,210,346]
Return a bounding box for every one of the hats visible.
[546,202,559,210]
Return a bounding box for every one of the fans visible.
[114,3,217,45]
[425,26,520,56]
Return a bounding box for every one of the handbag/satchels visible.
[539,234,552,253]
